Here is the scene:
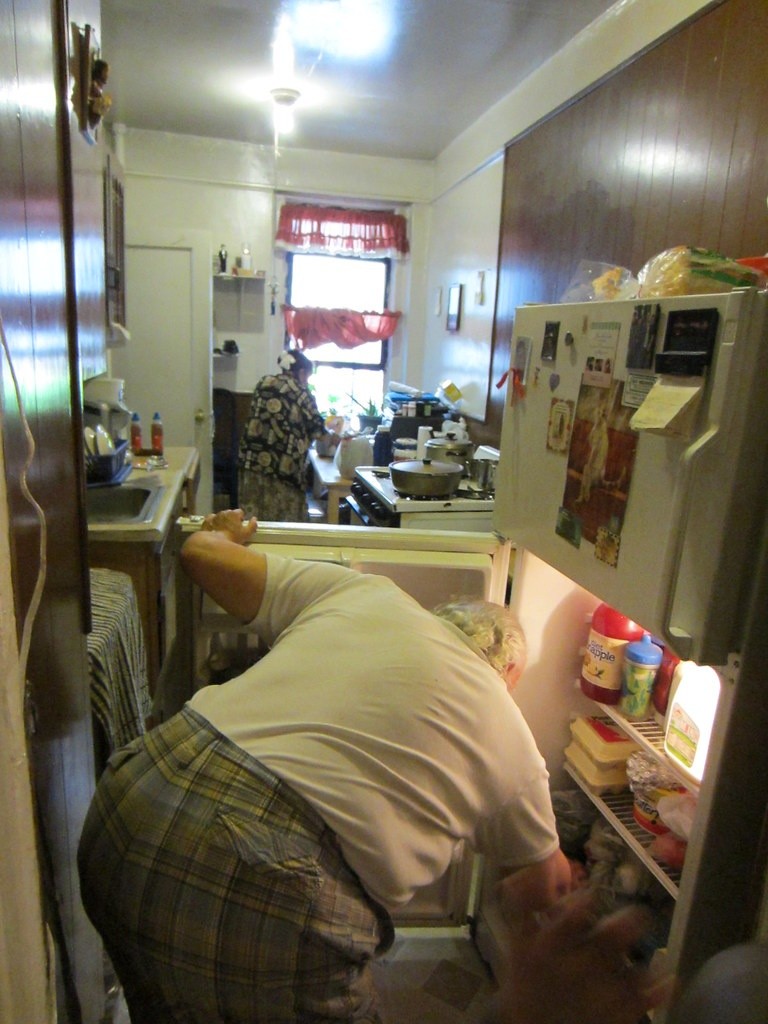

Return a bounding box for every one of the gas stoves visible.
[350,445,501,526]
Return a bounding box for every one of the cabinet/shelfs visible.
[88,453,206,729]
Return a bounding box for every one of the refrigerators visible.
[174,286,768,1024]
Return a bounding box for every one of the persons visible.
[236,350,342,523]
[77,509,573,1024]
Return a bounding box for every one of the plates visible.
[96,423,115,455]
[84,426,97,455]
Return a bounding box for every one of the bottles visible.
[151,412,164,456]
[579,601,645,706]
[131,412,142,455]
[619,635,663,721]
[416,426,433,460]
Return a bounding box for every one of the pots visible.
[463,457,499,492]
[424,432,475,476]
[388,458,464,497]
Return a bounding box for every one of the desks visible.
[304,449,358,524]
